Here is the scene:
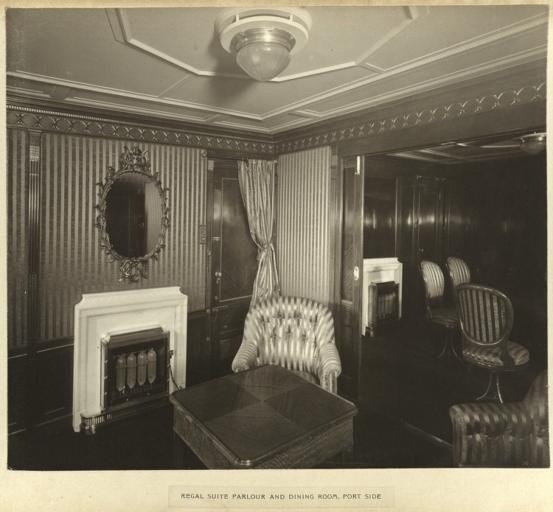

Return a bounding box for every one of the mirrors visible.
[93,144,171,281]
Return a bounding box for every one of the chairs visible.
[456,283,530,404]
[448,369,549,467]
[445,256,471,289]
[420,260,462,362]
[231,295,342,394]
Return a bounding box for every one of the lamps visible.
[512,132,547,155]
[215,7,313,82]
